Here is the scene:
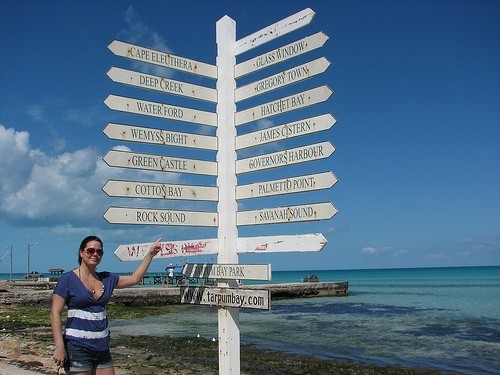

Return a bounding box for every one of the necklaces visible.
[81,270,95,293]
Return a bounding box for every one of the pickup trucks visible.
[24,271,44,279]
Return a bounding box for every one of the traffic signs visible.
[101,7,340,310]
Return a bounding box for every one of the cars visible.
[302,275,318,282]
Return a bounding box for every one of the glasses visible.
[81,248,104,256]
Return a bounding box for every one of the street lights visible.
[5,245,12,280]
[28,243,39,280]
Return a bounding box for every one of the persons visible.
[51,235,164,375]
